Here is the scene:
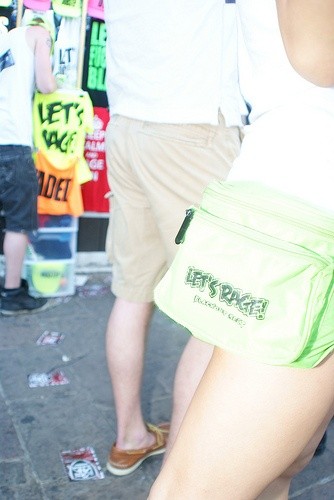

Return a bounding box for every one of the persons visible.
[145,0,334,498]
[0,17,51,315]
[101,0,242,475]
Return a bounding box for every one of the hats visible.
[26,13,55,54]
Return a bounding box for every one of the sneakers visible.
[1,278,50,316]
[108,420,176,478]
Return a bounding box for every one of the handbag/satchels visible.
[154,177,333,371]
